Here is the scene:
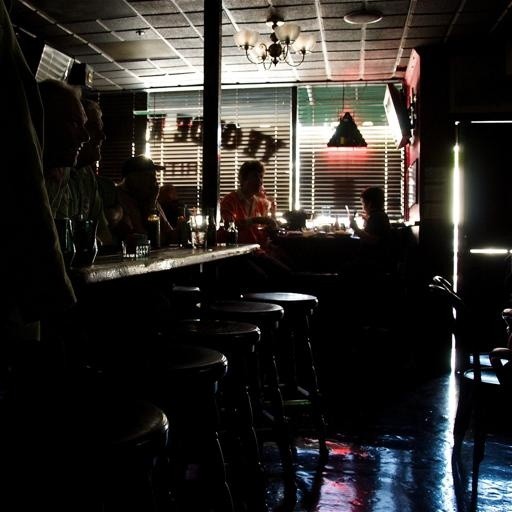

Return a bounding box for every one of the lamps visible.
[233,12,317,71]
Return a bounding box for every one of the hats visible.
[123,156,165,170]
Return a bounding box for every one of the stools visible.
[168,317,272,511]
[206,298,301,508]
[124,398,179,508]
[253,290,327,512]
[180,341,238,510]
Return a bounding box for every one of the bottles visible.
[225,219,239,246]
[178,205,218,252]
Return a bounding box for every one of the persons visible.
[347,187,391,243]
[220,161,274,276]
[37,78,102,275]
[105,154,192,237]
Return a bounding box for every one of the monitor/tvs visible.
[383,83,411,150]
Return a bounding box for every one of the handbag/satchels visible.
[257,226,284,263]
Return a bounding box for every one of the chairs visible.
[427,274,511,499]
